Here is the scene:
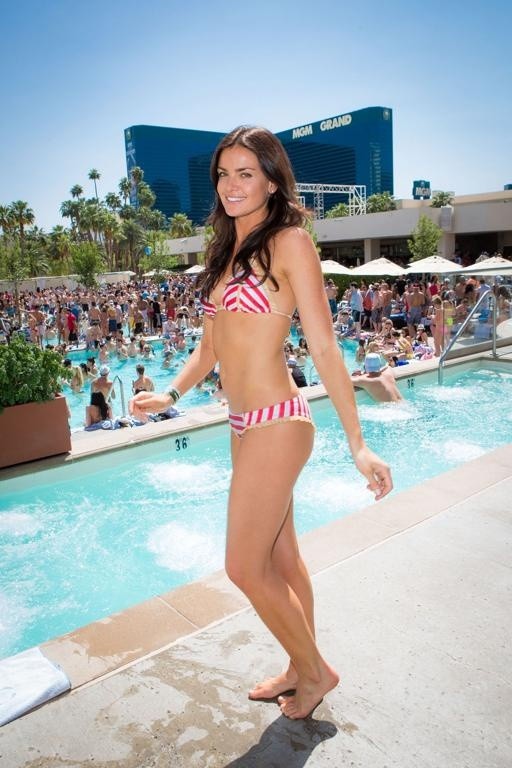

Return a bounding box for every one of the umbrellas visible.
[183,264,207,274]
[402,254,463,275]
[319,259,355,279]
[350,257,408,281]
[461,255,512,276]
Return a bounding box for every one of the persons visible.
[128,124,395,722]
[1,267,226,427]
[284,276,512,403]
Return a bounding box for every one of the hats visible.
[287,358,298,366]
[365,353,381,373]
[99,365,110,375]
[496,276,503,283]
[417,324,426,331]
